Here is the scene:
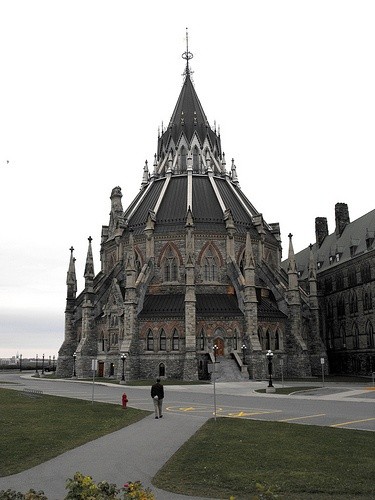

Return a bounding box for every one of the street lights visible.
[119,353,126,384]
[19,354,56,377]
[241,345,247,365]
[266,349,275,392]
[212,344,218,362]
[73,352,77,378]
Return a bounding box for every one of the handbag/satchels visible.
[154,395,158,400]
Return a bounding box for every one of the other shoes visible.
[160,415,164,418]
[155,416,158,419]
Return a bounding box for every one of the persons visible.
[151,378,164,419]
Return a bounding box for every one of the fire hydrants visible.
[122,393,128,409]
